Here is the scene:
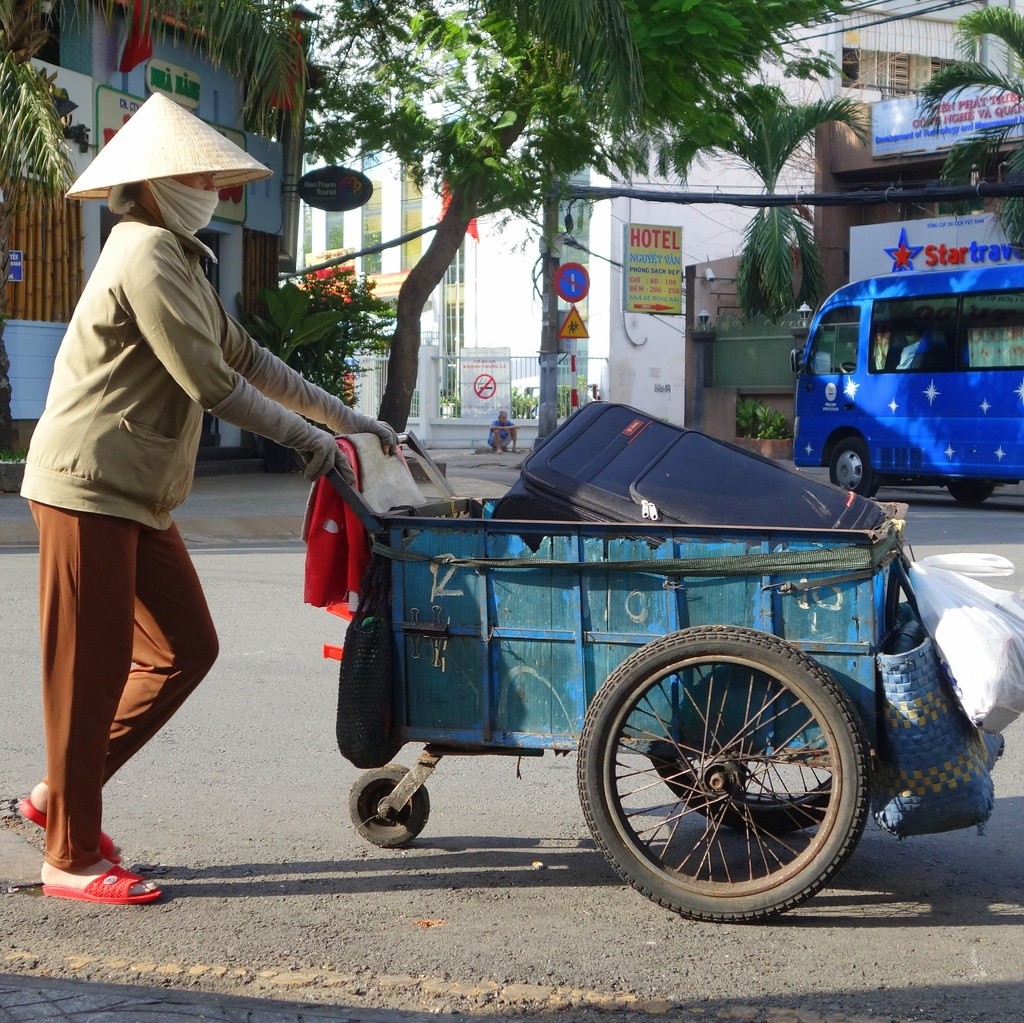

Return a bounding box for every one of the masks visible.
[148,177,220,263]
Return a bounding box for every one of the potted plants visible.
[231,281,346,472]
[0,448,30,493]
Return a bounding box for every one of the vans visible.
[784,265,1024,505]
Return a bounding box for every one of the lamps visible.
[796,301,813,326]
[699,308,710,330]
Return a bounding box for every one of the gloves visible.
[244,347,401,456]
[199,372,358,485]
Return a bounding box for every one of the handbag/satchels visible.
[908,550,1023,733]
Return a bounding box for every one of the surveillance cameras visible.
[705,267,716,281]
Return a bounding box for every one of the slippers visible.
[42,865,162,904]
[20,797,123,863]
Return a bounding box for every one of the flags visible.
[440,168,481,244]
[115,0,154,74]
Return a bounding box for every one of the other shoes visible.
[513,450,521,454]
[496,447,503,454]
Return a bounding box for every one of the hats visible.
[64,92,275,199]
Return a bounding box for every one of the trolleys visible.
[312,429,910,922]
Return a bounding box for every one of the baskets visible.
[863,555,1005,837]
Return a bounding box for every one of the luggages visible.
[490,400,884,529]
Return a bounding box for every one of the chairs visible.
[912,306,970,371]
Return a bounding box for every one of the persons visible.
[487,411,522,454]
[14,89,403,907]
[895,320,929,371]
